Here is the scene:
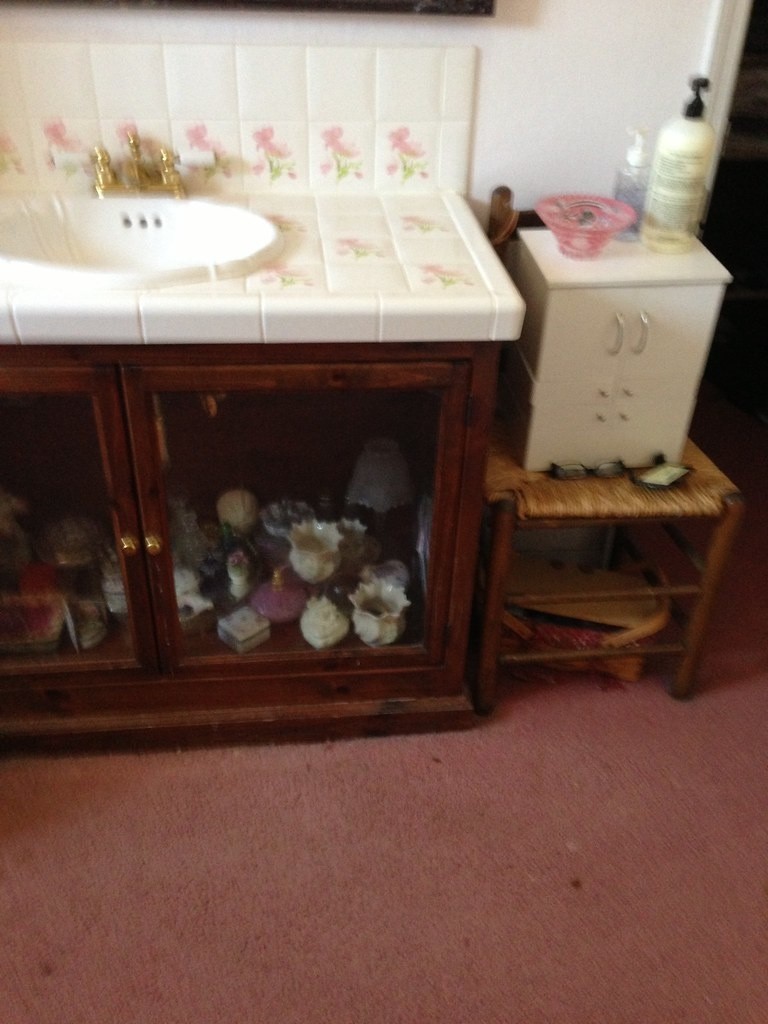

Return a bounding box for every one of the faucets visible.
[123,133,149,184]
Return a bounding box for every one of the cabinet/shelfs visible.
[0,343,501,759]
[504,224,735,477]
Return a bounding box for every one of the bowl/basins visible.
[534,195,637,260]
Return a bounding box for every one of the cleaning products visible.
[637,78,717,255]
[613,124,648,242]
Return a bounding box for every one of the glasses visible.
[552,459,625,480]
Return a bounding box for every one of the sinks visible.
[0,196,285,292]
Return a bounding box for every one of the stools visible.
[471,434,748,718]
